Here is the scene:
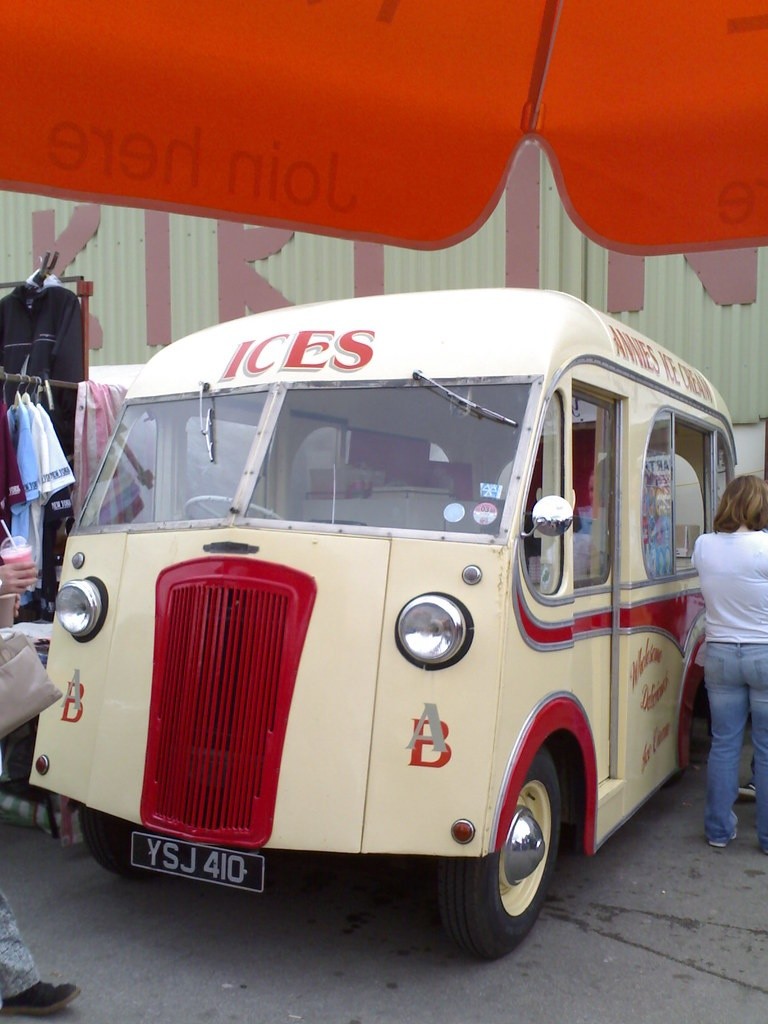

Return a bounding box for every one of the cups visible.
[0,545,34,584]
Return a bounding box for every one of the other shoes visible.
[738,782,756,801]
[709,832,736,847]
[1,981,81,1015]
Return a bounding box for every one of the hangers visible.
[2,372,42,408]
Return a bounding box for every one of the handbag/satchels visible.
[0,632,63,741]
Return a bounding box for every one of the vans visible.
[31,280,740,962]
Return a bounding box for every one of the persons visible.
[689,475,768,854]
[0,561,83,1014]
